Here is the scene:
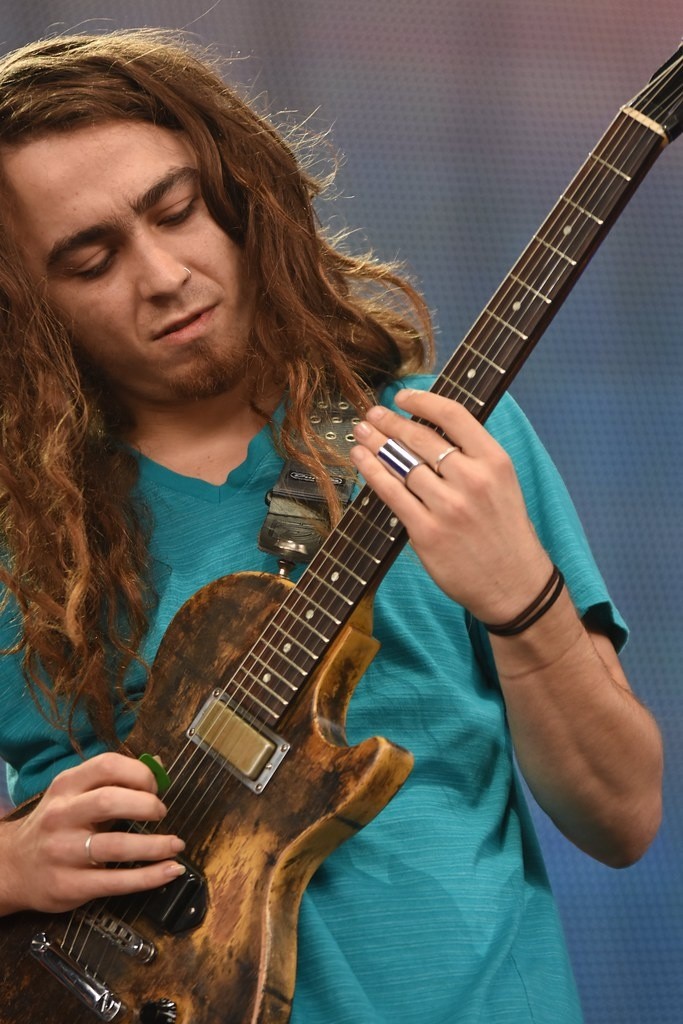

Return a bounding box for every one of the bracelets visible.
[483,563,564,638]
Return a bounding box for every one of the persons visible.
[0,29,665,1023]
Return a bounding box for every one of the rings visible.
[433,446,460,473]
[85,832,98,869]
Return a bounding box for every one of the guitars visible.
[0,45,682,1023]
